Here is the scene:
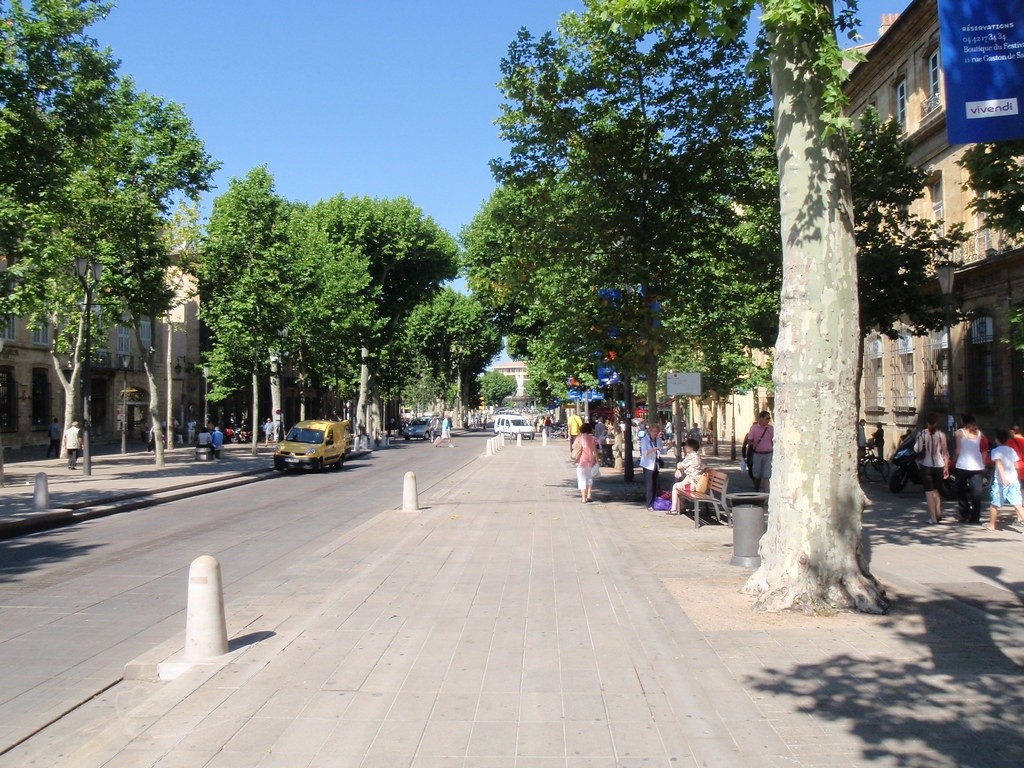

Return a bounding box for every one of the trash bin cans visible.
[726,491,770,567]
[596,443,616,458]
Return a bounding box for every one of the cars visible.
[401,417,442,440]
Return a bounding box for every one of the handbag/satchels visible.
[740,459,746,473]
[746,452,754,466]
[571,445,582,459]
[696,473,708,493]
[591,464,600,478]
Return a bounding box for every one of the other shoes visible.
[925,515,943,526]
[587,498,592,502]
[581,499,587,503]
[646,506,655,511]
[448,445,455,448]
[958,514,981,524]
[678,509,686,513]
[667,510,678,515]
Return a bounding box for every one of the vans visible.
[494,415,536,440]
[274,419,351,472]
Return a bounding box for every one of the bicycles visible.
[859,442,890,482]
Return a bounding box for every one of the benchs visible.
[195,445,226,461]
[676,468,732,527]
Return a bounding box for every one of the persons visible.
[858,418,884,461]
[431,415,455,449]
[913,408,1024,533]
[568,409,714,516]
[147,418,274,461]
[741,411,775,492]
[534,416,552,438]
[47,417,85,470]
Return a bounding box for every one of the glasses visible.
[767,418,771,419]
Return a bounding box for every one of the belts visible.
[753,451,772,454]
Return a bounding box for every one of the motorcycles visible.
[890,425,924,492]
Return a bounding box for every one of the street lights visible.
[204,363,210,428]
[934,260,959,416]
[119,351,133,455]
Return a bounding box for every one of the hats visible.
[681,439,698,446]
